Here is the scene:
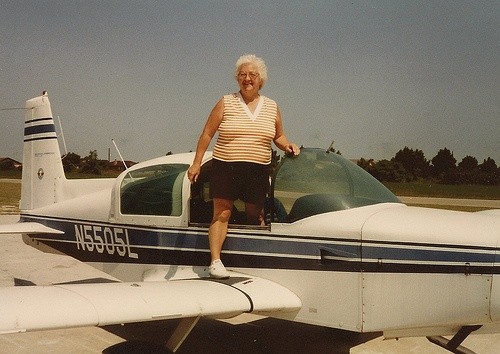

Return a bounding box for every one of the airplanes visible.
[0,90,500,354]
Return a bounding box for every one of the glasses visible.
[237,71,259,78]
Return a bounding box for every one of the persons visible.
[188,55,300,280]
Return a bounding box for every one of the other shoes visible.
[209,259,230,280]
